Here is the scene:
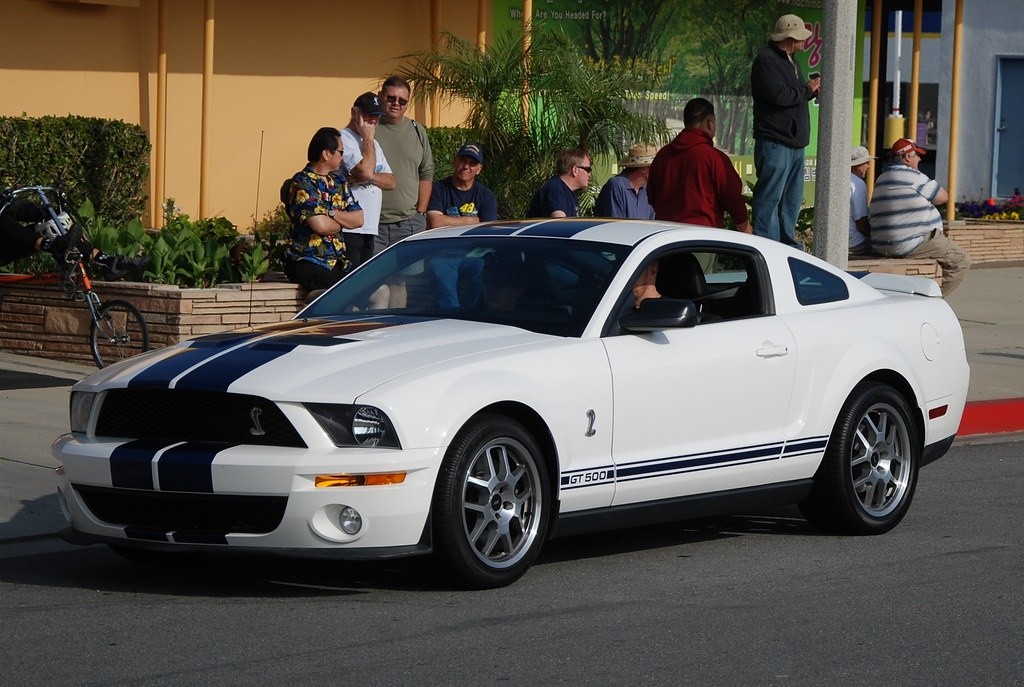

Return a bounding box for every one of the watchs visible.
[328,209,336,220]
[417,211,426,217]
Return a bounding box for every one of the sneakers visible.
[106,254,152,281]
[53,224,82,265]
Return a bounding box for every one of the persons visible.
[869,138,971,300]
[593,143,661,221]
[520,149,592,218]
[848,146,871,256]
[645,98,753,275]
[749,14,820,253]
[425,143,498,311]
[619,257,674,308]
[363,78,435,313]
[284,127,389,316]
[338,92,396,274]
[0,194,152,284]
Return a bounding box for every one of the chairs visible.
[455,251,534,309]
[657,250,706,324]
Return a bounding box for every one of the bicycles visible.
[0,185,150,370]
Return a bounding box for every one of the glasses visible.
[384,93,408,105]
[577,165,592,174]
[328,149,344,157]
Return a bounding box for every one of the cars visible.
[50,217,971,590]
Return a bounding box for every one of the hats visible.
[850,146,879,167]
[353,92,387,117]
[617,142,661,166]
[770,14,813,43]
[892,137,926,157]
[457,144,483,163]
